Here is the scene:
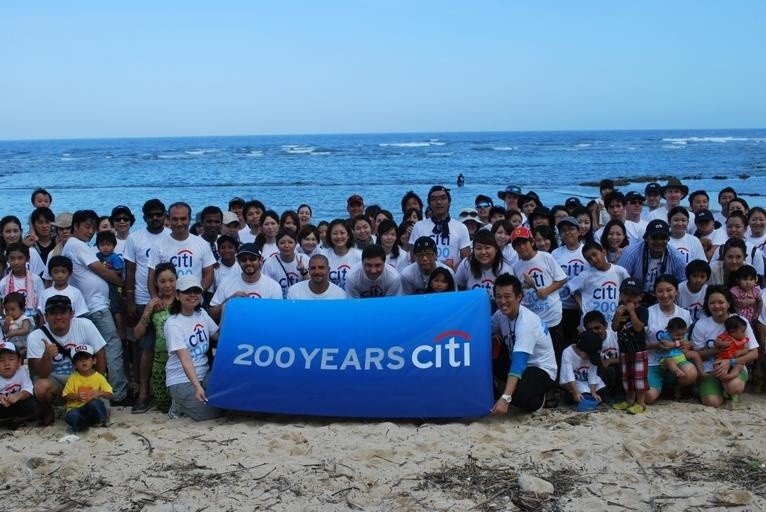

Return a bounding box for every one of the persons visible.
[0,172,765,435]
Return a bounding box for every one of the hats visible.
[46,295,71,311]
[50,196,260,294]
[624,177,713,235]
[70,344,95,364]
[347,185,582,251]
[0,341,22,359]
[576,331,602,365]
[619,278,644,295]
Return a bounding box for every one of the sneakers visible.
[532,388,738,417]
[26,383,183,427]
